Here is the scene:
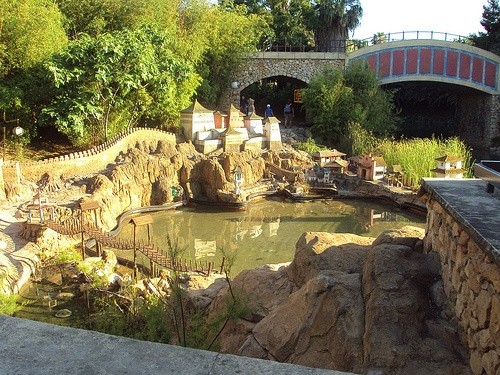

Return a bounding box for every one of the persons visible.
[240,94,295,129]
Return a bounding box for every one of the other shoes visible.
[284,126,287,128]
[288,126,292,128]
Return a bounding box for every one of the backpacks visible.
[284,103,292,113]
